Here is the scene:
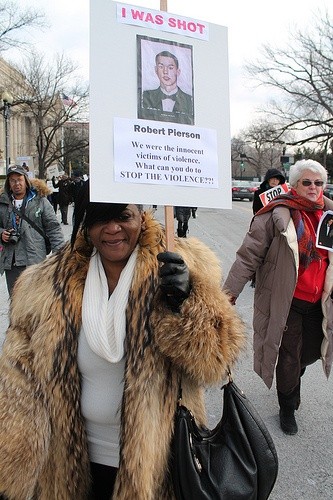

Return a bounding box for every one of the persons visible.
[222,159,333,436]
[0,199,247,500]
[142,51,194,115]
[319,219,333,248]
[52,174,74,225]
[0,165,65,303]
[173,206,197,238]
[253,168,285,215]
[72,173,89,225]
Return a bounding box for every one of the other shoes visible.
[61,222,69,225]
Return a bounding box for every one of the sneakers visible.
[280,407,298,436]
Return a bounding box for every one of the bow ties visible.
[160,92,178,100]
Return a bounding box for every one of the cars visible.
[323,184,333,202]
[231,180,262,201]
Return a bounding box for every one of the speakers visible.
[280,157,289,162]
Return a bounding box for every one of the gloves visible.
[156,252,190,313]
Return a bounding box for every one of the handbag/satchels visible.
[169,343,279,500]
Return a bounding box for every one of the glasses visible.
[297,180,324,186]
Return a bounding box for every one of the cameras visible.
[7,228,24,245]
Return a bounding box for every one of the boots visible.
[177,229,183,237]
[182,225,188,238]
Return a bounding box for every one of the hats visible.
[6,165,26,176]
[77,177,129,232]
